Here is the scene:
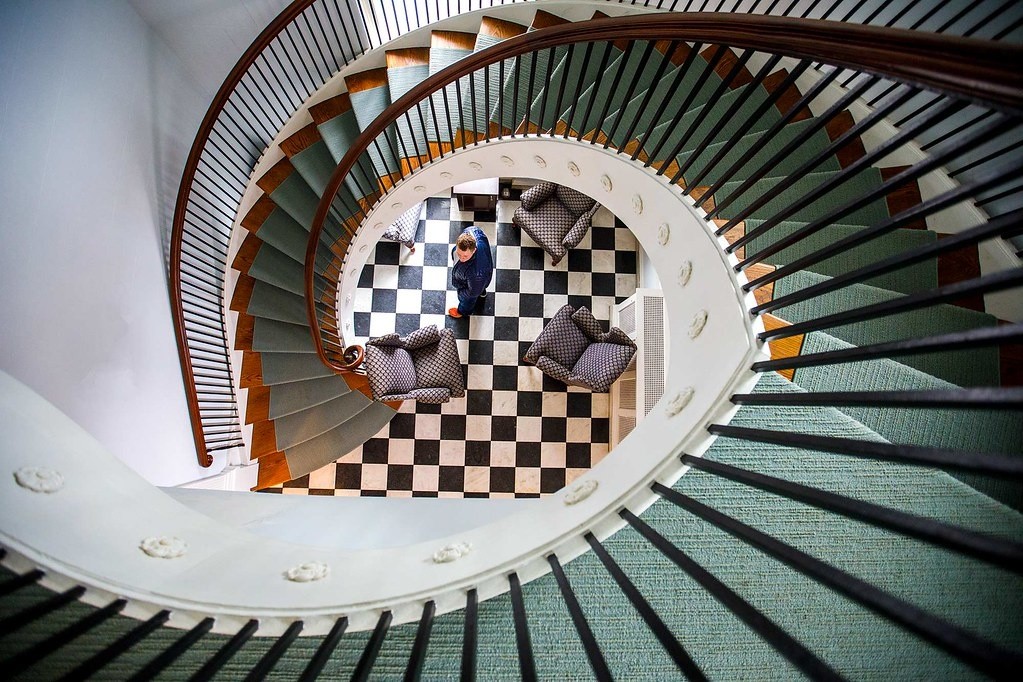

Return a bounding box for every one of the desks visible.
[452,176,499,201]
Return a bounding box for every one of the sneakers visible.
[480,289,487,297]
[449,307,462,317]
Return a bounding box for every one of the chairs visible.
[381,200,423,254]
[522,304,638,394]
[365,324,466,404]
[512,181,602,266]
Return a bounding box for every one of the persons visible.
[449,226,494,320]
